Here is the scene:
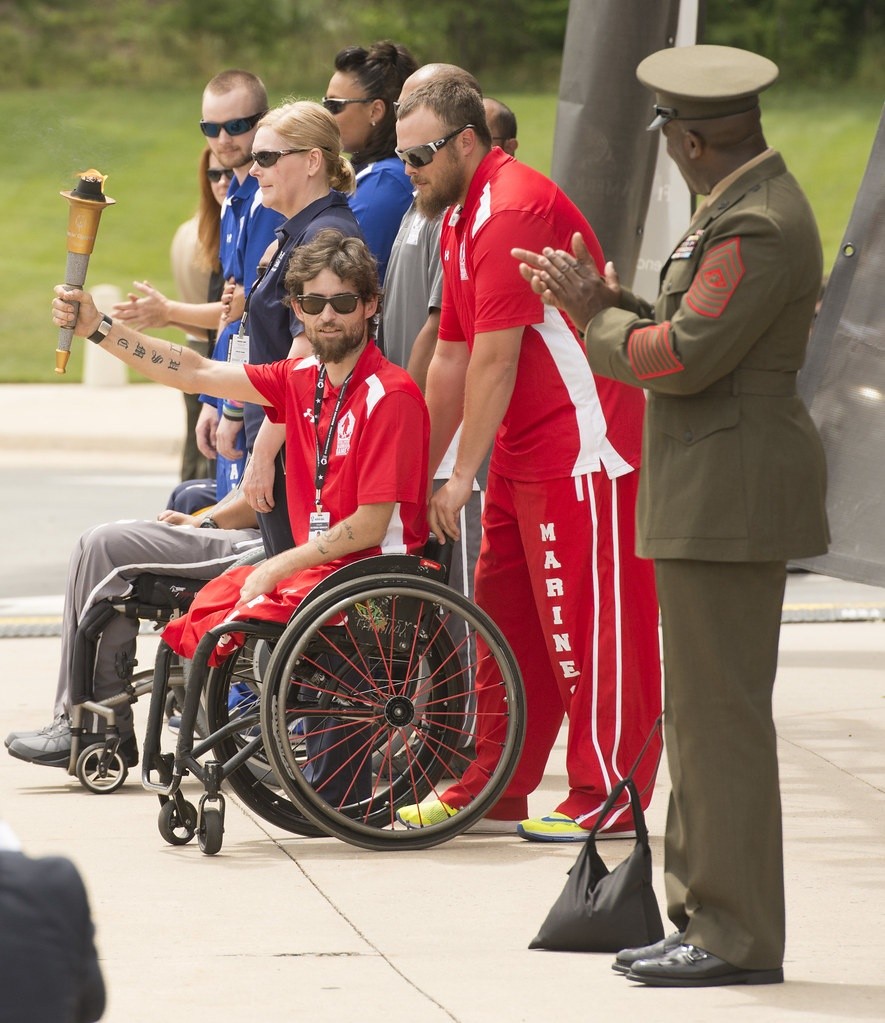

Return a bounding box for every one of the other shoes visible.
[240,722,307,744]
[168,715,202,740]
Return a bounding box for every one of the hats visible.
[635,44,779,131]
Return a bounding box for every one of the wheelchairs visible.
[66,497,529,856]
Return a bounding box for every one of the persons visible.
[481,96,522,161]
[4,39,420,813]
[377,62,487,782]
[393,78,664,840]
[511,44,832,988]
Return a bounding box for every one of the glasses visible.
[394,125,475,168]
[297,295,361,314]
[198,112,265,138]
[256,266,267,275]
[251,147,330,168]
[322,97,388,114]
[206,169,234,183]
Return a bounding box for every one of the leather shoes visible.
[625,943,784,986]
[611,928,688,973]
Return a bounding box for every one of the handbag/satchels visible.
[528,778,665,952]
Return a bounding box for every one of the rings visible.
[257,499,265,502]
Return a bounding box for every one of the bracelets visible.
[87,311,113,344]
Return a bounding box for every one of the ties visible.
[690,200,708,227]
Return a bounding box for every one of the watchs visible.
[200,514,218,528]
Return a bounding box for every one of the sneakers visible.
[8,724,138,769]
[518,811,648,842]
[4,719,64,748]
[395,799,524,834]
